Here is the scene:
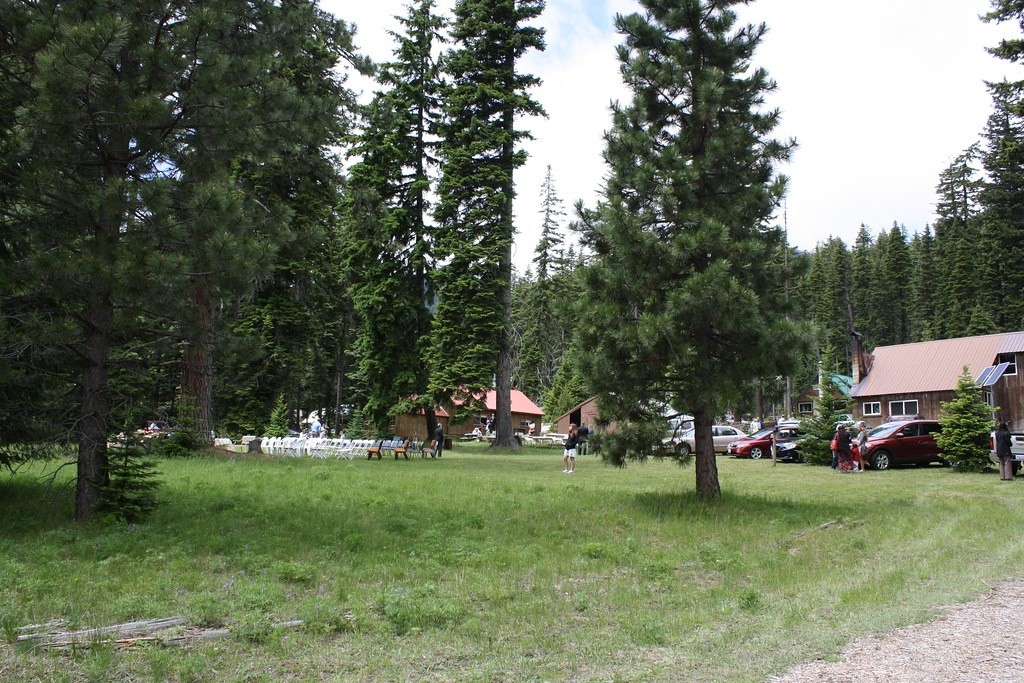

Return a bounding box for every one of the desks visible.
[517,434,569,445]
[486,435,496,446]
[464,434,478,442]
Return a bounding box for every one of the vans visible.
[302,407,350,431]
[649,415,696,455]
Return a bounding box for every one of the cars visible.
[770,413,874,463]
[661,425,746,456]
[847,419,960,471]
[726,426,806,461]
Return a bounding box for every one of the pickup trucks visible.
[989,430,1024,476]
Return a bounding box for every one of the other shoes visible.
[567,470,574,473]
[562,470,568,472]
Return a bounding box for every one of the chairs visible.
[232,436,438,460]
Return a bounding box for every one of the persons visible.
[995,422,1013,481]
[528,420,536,436]
[562,424,579,474]
[472,413,496,442]
[300,425,346,440]
[778,414,793,430]
[742,417,761,434]
[434,423,443,457]
[830,422,868,474]
[577,421,594,455]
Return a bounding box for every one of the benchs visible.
[460,437,476,442]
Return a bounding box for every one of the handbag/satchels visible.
[831,437,838,450]
